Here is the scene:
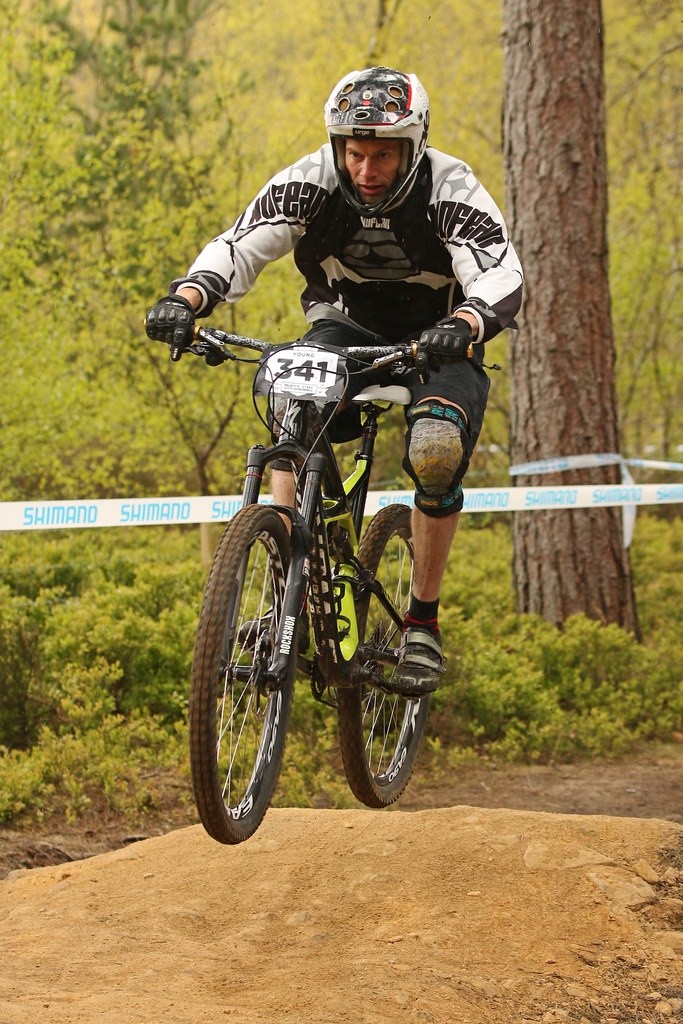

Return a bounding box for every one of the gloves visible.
[414,316,473,385]
[146,292,197,362]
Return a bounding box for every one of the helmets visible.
[324,67,429,219]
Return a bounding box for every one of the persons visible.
[142,65,525,698]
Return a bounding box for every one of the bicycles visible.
[137,301,481,847]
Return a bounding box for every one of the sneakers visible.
[239,606,310,654]
[390,627,446,693]
